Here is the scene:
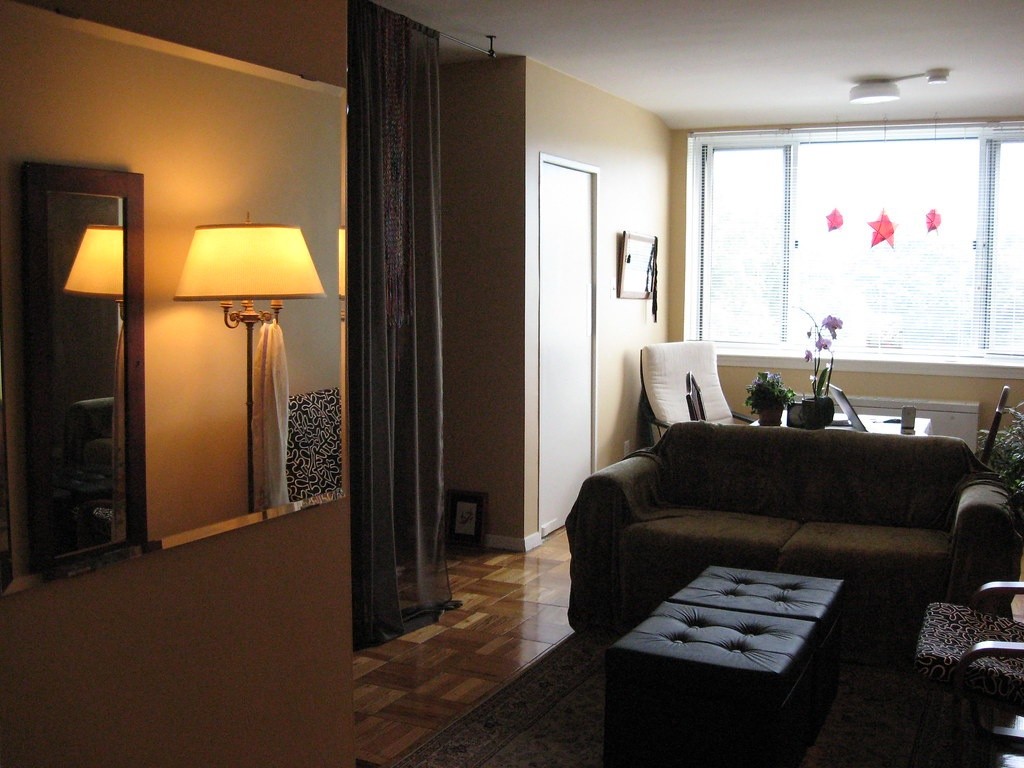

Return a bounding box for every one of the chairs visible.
[640,339,756,447]
[915,580,1024,746]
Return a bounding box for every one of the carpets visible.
[382,623,997,768]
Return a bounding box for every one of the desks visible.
[749,410,932,436]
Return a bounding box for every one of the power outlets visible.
[624,440,630,456]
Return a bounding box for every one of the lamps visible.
[63,222,125,321]
[168,210,327,518]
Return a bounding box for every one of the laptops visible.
[825,382,868,434]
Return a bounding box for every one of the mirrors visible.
[18,159,163,586]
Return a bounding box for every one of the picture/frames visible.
[616,230,655,300]
[446,489,489,549]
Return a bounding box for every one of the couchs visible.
[567,420,1024,668]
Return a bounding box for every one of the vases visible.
[756,400,784,426]
[801,398,825,432]
[822,396,835,425]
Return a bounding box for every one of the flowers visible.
[744,370,797,413]
[793,302,844,397]
[804,332,831,399]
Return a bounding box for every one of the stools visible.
[604,600,818,768]
[669,564,847,744]
